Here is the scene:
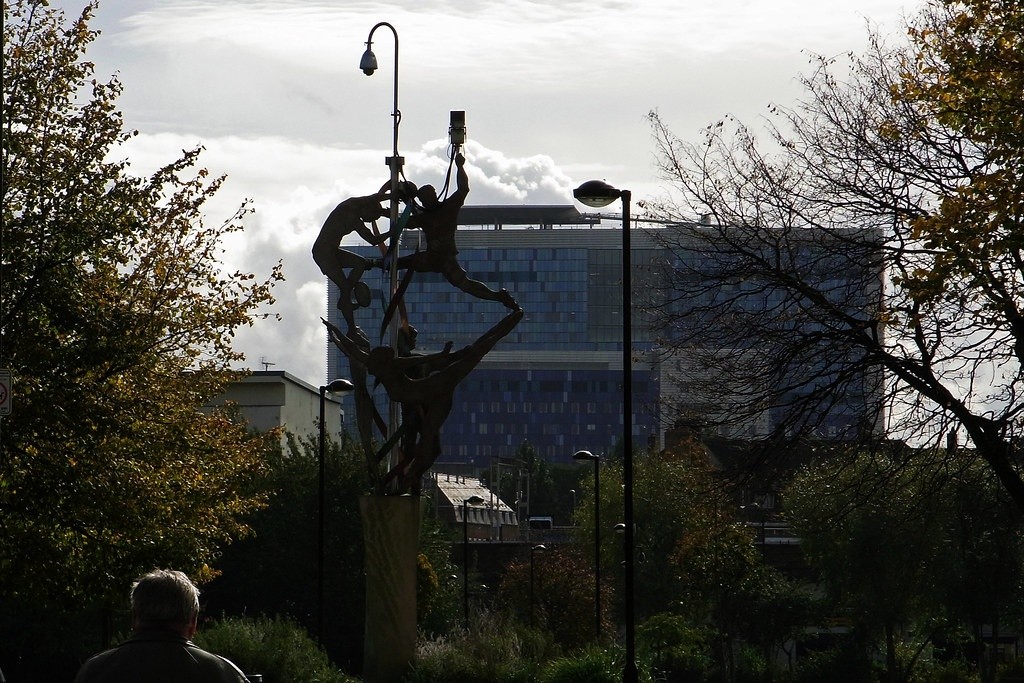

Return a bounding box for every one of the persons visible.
[312,194,399,336]
[73,571,249,683]
[322,307,524,496]
[371,153,519,311]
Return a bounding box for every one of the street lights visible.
[360,19,400,495]
[900,618,962,683]
[571,450,601,640]
[571,180,639,683]
[750,502,766,563]
[530,545,547,629]
[317,378,355,652]
[463,495,484,630]
